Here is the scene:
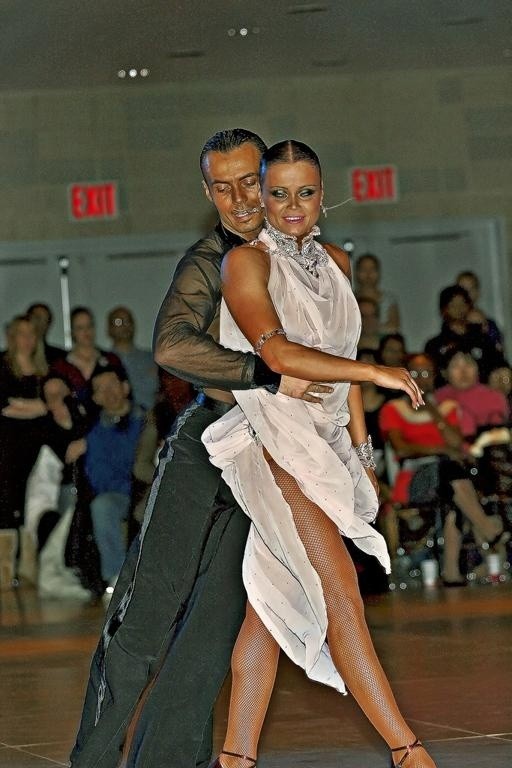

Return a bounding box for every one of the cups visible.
[487,554,503,584]
[421,559,438,587]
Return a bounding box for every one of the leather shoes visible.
[443,530,511,587]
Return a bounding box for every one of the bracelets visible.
[357,434,377,471]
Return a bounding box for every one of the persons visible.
[0,303,197,605]
[199,140,438,768]
[68,128,333,768]
[346,254,509,588]
